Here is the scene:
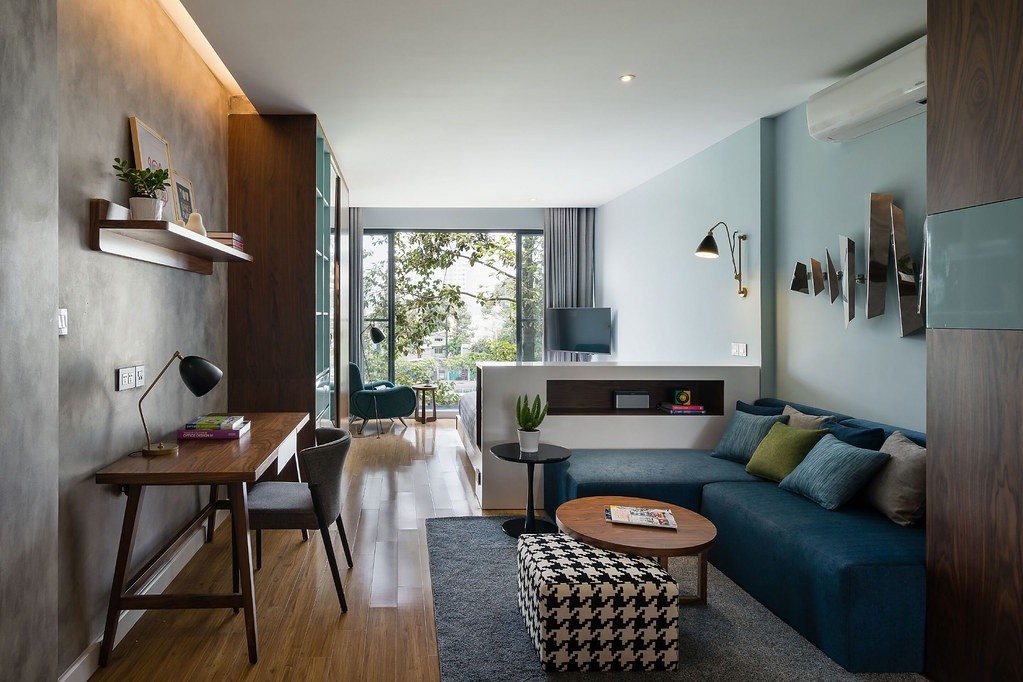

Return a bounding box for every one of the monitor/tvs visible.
[545,307,612,355]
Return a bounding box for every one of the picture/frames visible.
[130,115,196,224]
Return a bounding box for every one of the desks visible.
[413,384,436,424]
[96,409,310,669]
[555,496,717,607]
[489,443,571,539]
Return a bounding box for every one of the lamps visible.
[360,322,385,445]
[137,351,224,456]
[694,221,747,299]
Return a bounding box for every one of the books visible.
[177,416,251,439]
[207,231,243,252]
[604,505,677,528]
[661,402,706,414]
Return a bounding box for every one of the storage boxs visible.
[611,390,650,412]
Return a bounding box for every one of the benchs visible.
[517,533,679,671]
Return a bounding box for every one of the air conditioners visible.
[806,34,928,144]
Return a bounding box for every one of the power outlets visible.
[117,366,144,389]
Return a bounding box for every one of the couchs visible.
[703,418,928,675]
[542,395,854,527]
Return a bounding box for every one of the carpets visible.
[425,514,930,682]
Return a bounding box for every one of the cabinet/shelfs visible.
[226,111,336,432]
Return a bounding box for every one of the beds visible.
[456,390,483,474]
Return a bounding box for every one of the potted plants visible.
[514,393,549,453]
[112,158,171,220]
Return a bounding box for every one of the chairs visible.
[232,427,354,615]
[348,360,416,434]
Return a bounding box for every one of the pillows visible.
[709,400,927,530]
